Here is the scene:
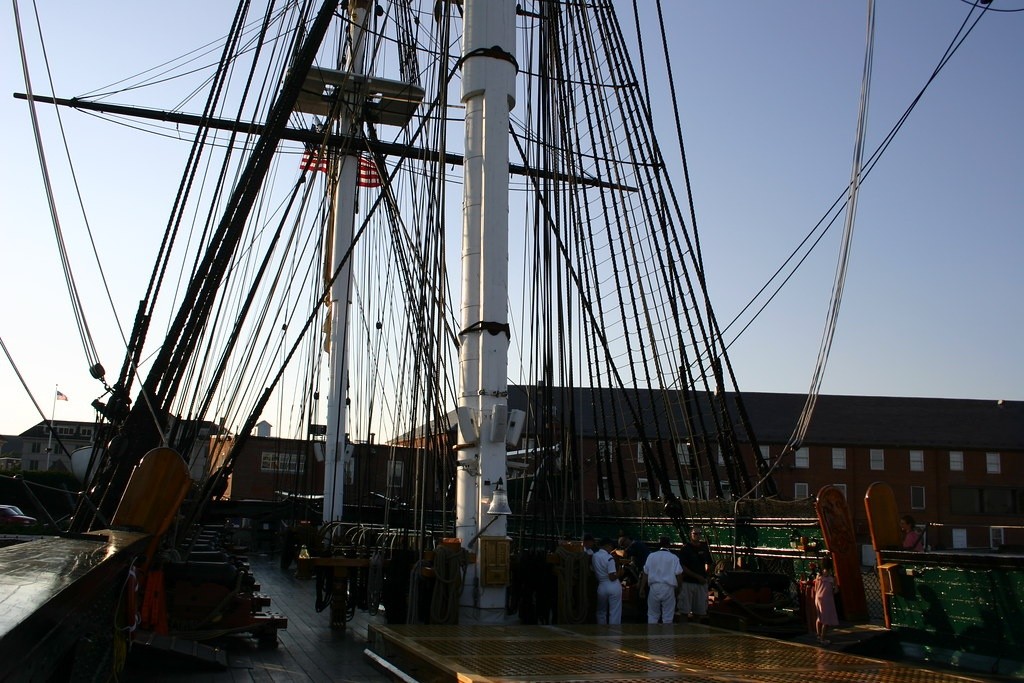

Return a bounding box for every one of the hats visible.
[600,537,613,546]
[584,534,594,541]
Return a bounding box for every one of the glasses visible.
[692,532,702,535]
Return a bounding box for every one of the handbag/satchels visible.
[715,558,728,576]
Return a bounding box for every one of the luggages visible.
[742,549,759,571]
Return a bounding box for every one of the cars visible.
[0,505,40,535]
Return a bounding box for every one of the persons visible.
[901,515,924,551]
[814,557,839,645]
[582,525,713,624]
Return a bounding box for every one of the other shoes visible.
[819,640,832,646]
[816,635,820,640]
